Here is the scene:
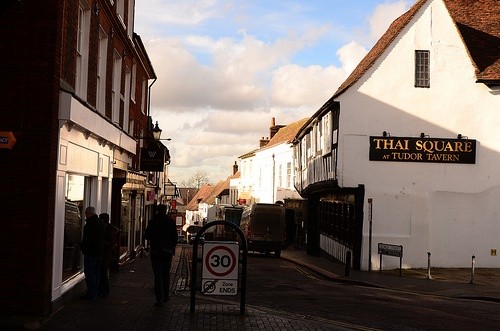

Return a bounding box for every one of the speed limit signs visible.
[203,242,238,295]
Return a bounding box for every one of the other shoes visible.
[80,289,114,300]
[155,297,169,306]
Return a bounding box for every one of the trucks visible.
[205,204,243,240]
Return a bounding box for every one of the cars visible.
[189,233,205,244]
[179,235,187,243]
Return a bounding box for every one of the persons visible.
[80,206,104,309]
[144,204,178,304]
[97,212,119,299]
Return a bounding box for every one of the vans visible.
[235,203,288,256]
